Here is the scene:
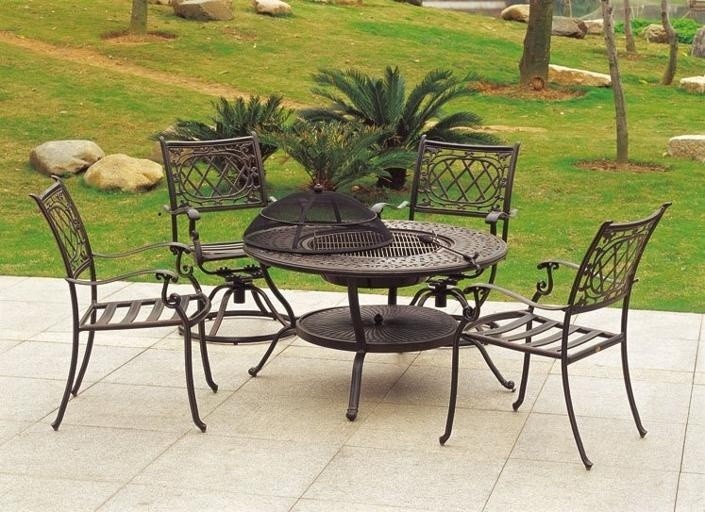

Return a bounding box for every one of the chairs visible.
[438,200,673,470]
[389,134,522,345]
[160,130,298,344]
[29,173,224,434]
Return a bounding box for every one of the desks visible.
[243,221,507,422]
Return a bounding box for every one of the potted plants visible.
[171,94,288,186]
[294,65,500,191]
